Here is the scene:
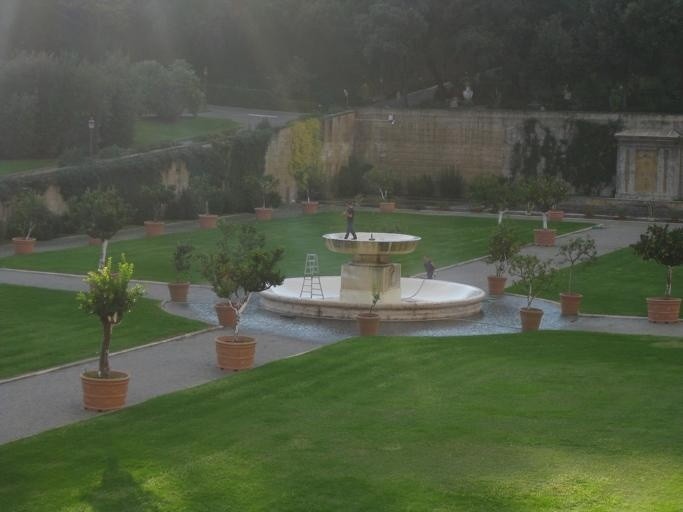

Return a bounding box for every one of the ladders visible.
[299,249,324,299]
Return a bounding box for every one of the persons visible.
[342,200,357,240]
[423,255,436,279]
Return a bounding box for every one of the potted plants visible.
[521,178,567,250]
[7,188,51,254]
[165,219,286,371]
[354,283,384,338]
[628,222,683,325]
[363,167,400,211]
[64,170,320,245]
[479,229,600,330]
[76,254,144,413]
